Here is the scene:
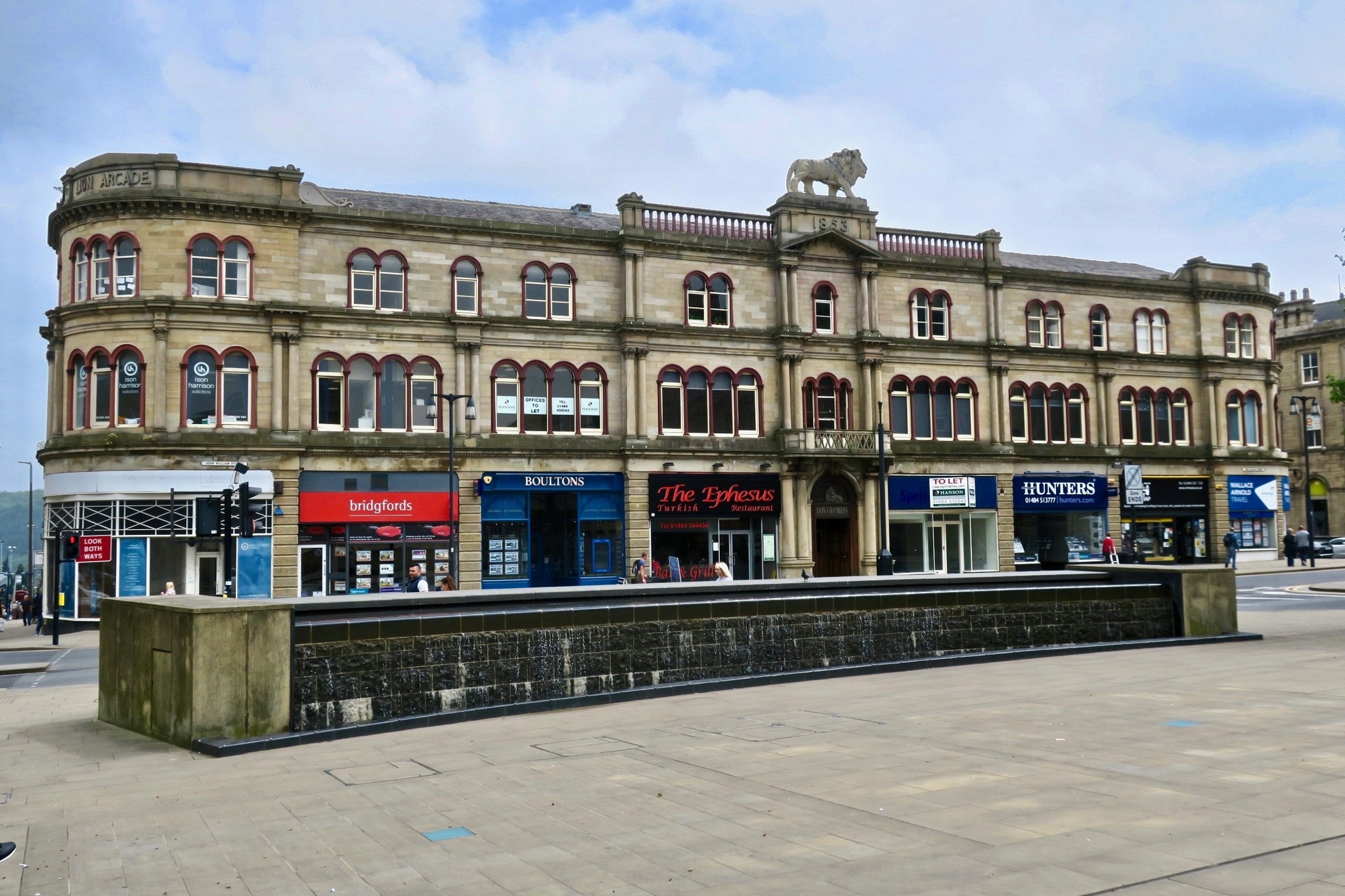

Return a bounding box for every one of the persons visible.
[714,562,734,582]
[631,552,647,583]
[440,575,457,591]
[1282,525,1310,567]
[1222,528,1239,569]
[1102,532,1115,564]
[0,842,17,862]
[406,563,429,593]
[160,581,176,595]
[1,586,43,635]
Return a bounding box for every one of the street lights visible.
[424,392,476,583]
[0,540,5,573]
[7,546,17,573]
[17,461,34,614]
[1288,396,1323,567]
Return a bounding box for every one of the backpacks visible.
[631,559,642,574]
[1224,533,1234,547]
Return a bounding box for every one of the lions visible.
[787,147,868,198]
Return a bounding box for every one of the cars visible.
[1308,539,1334,559]
[1328,537,1345,559]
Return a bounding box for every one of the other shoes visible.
[1302,561,1306,566]
[1233,567,1237,570]
[1225,563,1228,568]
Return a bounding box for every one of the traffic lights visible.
[63,532,80,559]
[238,482,263,539]
